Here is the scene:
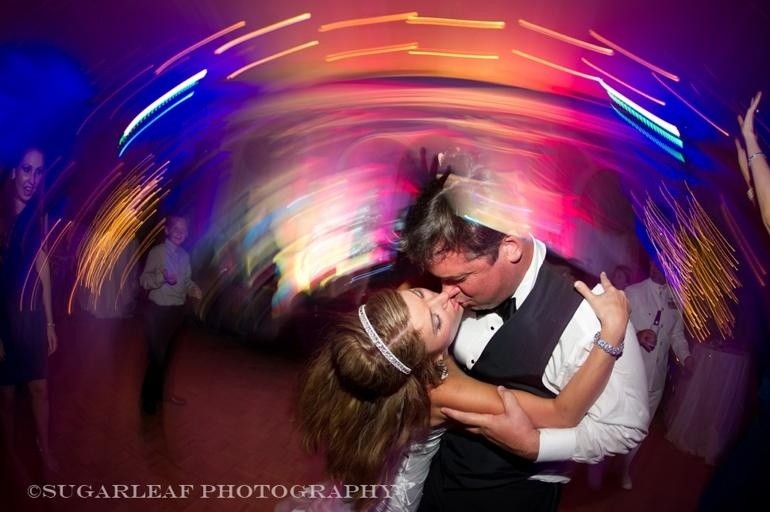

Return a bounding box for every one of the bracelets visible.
[594,331,624,356]
[47,322,55,327]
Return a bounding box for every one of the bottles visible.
[648,309,662,350]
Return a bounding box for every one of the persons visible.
[609,94,770,512]
[277,269,631,512]
[1,145,60,470]
[399,176,652,511]
[139,215,203,440]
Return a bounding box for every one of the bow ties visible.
[474,296,517,321]
